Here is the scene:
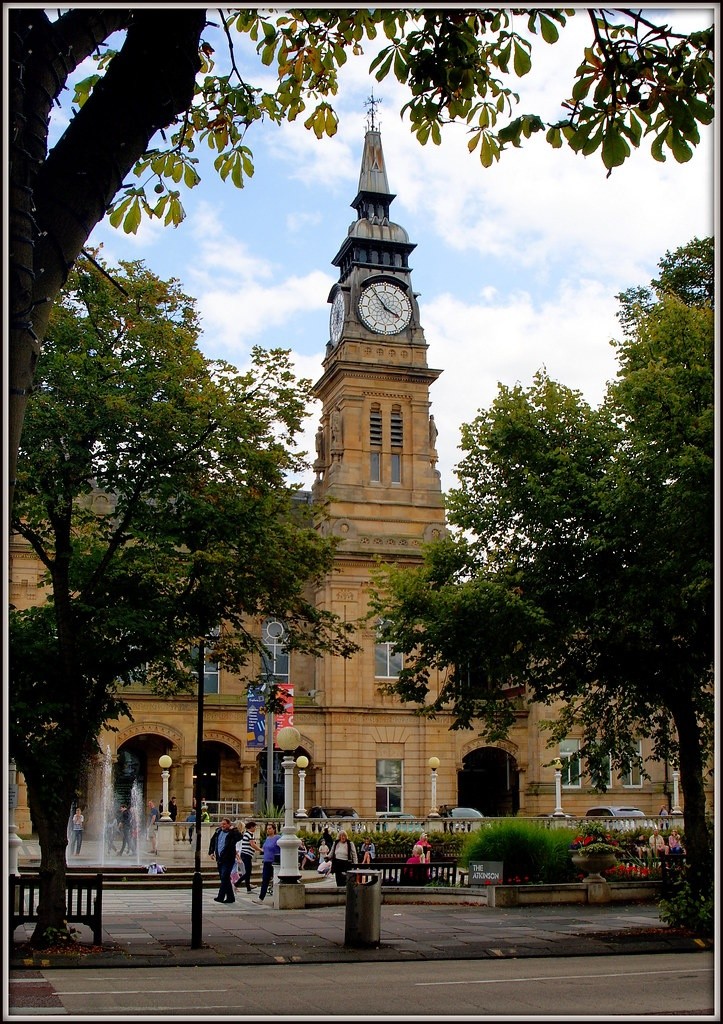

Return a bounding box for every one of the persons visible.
[69,808,85,857]
[105,797,210,854]
[208,819,375,905]
[634,805,685,859]
[404,833,432,881]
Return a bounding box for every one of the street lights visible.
[554,757,566,818]
[278,727,303,882]
[159,755,174,821]
[191,635,222,946]
[428,757,440,819]
[296,754,309,819]
[672,758,682,816]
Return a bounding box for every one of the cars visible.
[377,812,422,833]
[308,806,364,834]
[583,806,658,830]
[447,808,488,834]
[535,812,580,829]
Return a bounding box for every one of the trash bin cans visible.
[344,871,382,948]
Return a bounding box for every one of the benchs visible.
[9,873,103,944]
[348,843,462,886]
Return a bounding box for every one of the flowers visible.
[568,820,623,858]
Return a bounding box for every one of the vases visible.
[568,849,617,883]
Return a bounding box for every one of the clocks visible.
[330,290,346,347]
[357,281,412,335]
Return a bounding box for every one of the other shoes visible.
[232,883,238,897]
[247,890,257,894]
[253,898,264,905]
[213,897,235,904]
[250,884,257,889]
